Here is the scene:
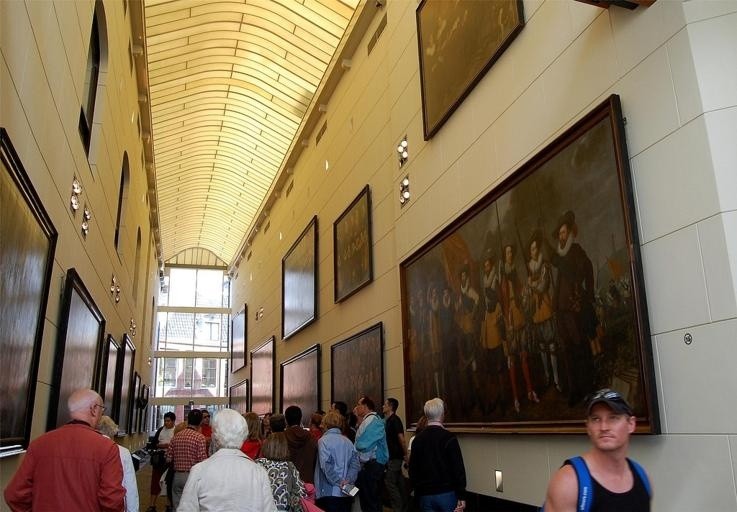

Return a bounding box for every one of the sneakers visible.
[512,398,521,413]
[544,376,551,387]
[553,382,562,393]
[527,391,540,404]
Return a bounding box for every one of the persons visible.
[406,398,467,512]
[328,401,348,433]
[95,415,140,512]
[284,405,316,484]
[268,414,285,433]
[406,415,428,466]
[239,412,264,460]
[146,411,176,512]
[412,210,602,417]
[4,388,127,512]
[383,397,406,512]
[354,397,389,512]
[261,412,272,437]
[541,388,654,512]
[308,414,323,443]
[313,412,360,512]
[198,409,213,459]
[166,409,207,512]
[254,431,306,512]
[176,408,278,512]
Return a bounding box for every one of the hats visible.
[557,211,576,225]
[585,387,631,415]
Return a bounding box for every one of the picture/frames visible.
[128,371,142,436]
[279,213,319,344]
[278,342,323,428]
[227,377,250,416]
[329,321,385,429]
[228,301,248,375]
[113,333,138,439]
[0,125,60,461]
[398,92,664,438]
[248,335,277,419]
[45,266,107,436]
[139,382,151,435]
[332,183,374,305]
[415,0,526,142]
[99,332,124,425]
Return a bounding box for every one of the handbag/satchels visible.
[355,415,383,470]
[285,461,303,512]
[149,450,168,471]
[401,460,410,479]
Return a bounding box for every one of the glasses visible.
[203,415,210,418]
[97,403,107,413]
